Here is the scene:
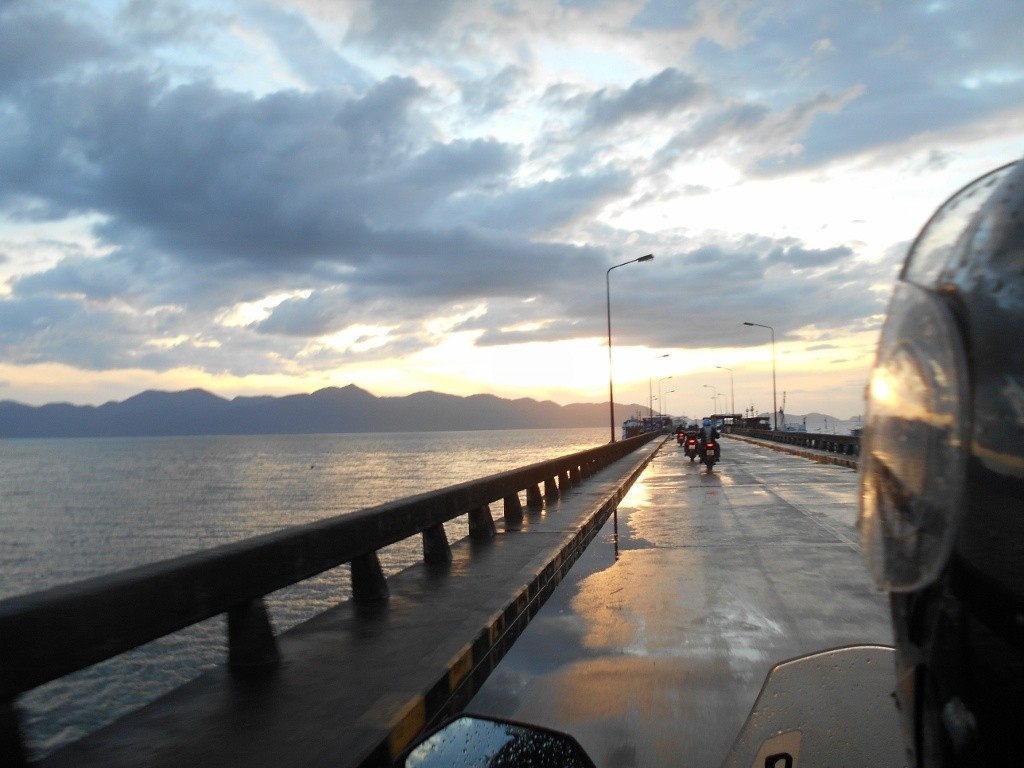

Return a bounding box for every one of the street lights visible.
[744,321,779,431]
[704,385,717,426]
[648,353,669,432]
[604,254,655,443]
[657,376,675,429]
[716,365,735,426]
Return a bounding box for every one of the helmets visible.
[703,419,712,426]
[855,161,1024,768]
[687,421,696,426]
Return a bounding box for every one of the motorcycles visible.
[697,432,720,470]
[684,430,701,463]
[676,428,686,446]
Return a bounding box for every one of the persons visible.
[698,420,721,463]
[676,423,699,456]
[861,158,1024,768]
